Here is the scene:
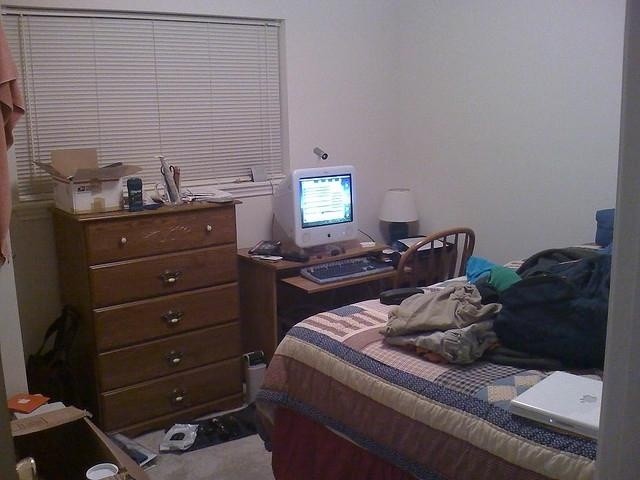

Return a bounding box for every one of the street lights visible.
[27,345,82,408]
[479,247,612,356]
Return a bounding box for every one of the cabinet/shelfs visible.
[126,178,144,212]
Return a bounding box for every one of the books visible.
[10,404,151,480]
[32,147,143,216]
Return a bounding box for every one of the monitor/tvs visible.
[8,392,52,416]
[188,188,235,204]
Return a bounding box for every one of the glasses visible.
[376,188,419,251]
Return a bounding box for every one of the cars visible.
[155,174,184,207]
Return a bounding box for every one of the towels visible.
[244,351,267,405]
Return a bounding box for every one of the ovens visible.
[271,241,614,479]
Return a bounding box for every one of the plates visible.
[46,204,244,441]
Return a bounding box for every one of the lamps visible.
[393,226,477,289]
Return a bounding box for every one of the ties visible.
[273,165,360,258]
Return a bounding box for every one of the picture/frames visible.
[236,239,413,368]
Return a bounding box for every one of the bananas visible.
[299,256,394,284]
